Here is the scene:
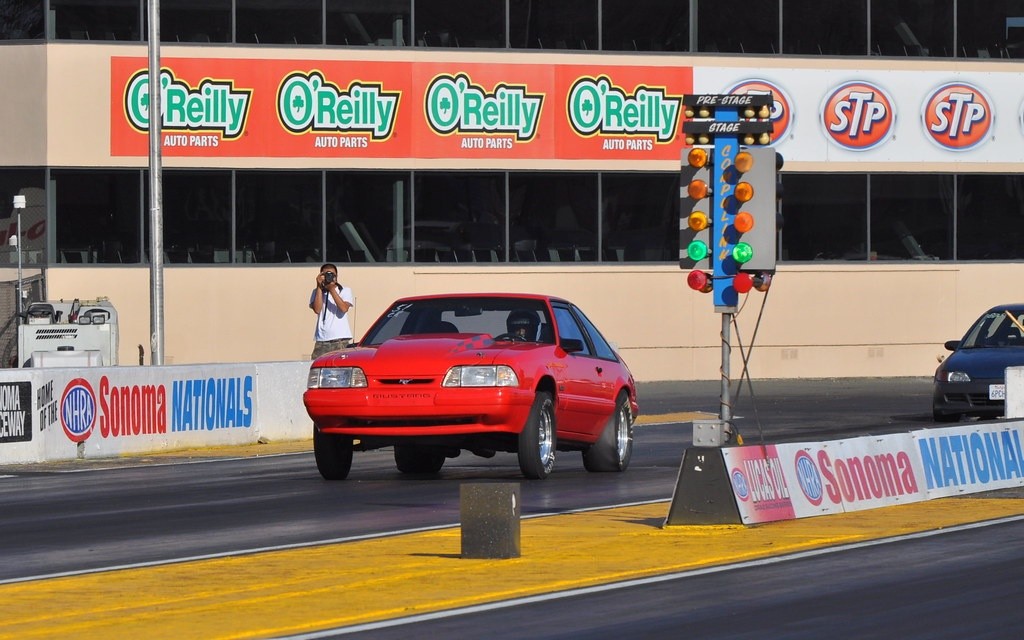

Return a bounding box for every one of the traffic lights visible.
[680,147,784,295]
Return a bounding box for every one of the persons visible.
[511,320,530,343]
[309,263,354,359]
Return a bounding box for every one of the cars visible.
[931,302,1024,424]
[301,291,639,477]
[388,218,507,263]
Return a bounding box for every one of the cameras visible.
[322,271,335,286]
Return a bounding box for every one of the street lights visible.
[9,195,28,323]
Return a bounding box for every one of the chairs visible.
[419,321,459,333]
[992,327,1022,346]
